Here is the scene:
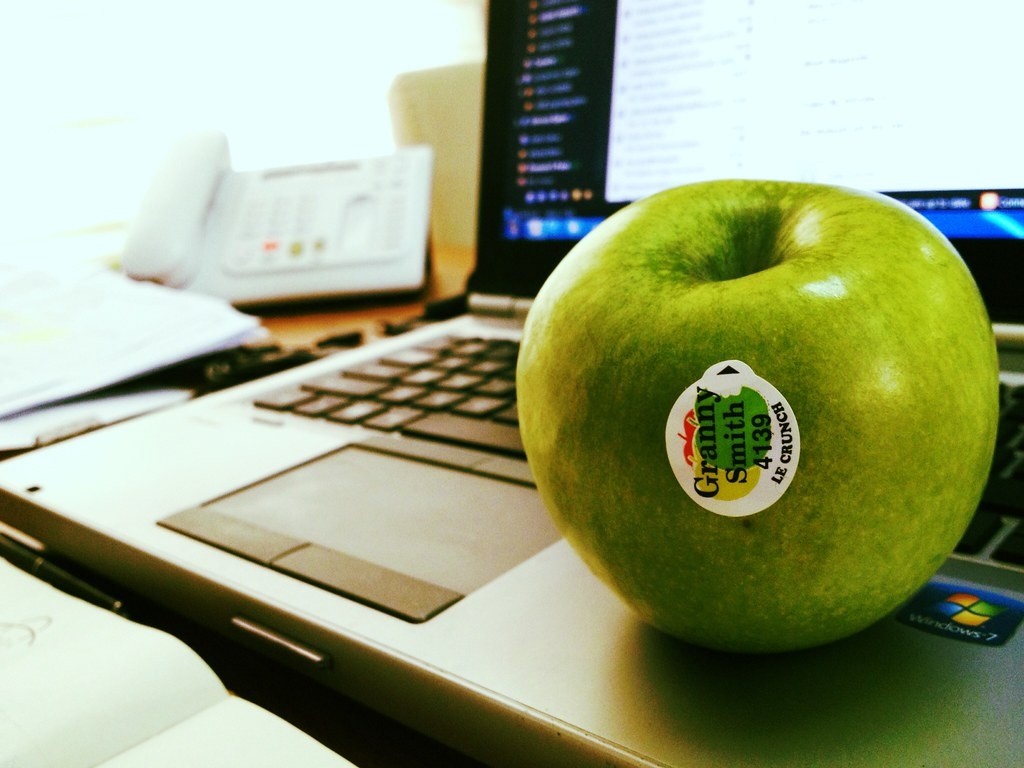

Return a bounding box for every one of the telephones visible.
[114,122,437,307]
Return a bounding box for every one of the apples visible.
[513,179,999,654]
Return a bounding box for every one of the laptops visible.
[0,0,1024,768]
